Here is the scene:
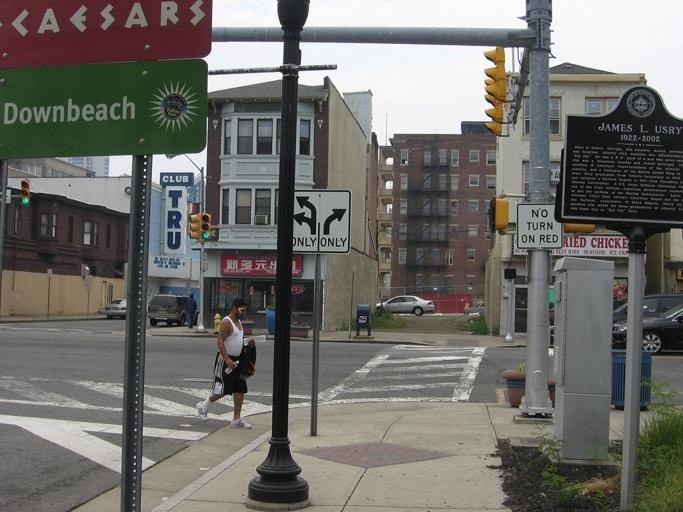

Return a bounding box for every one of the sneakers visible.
[231,419,251,429]
[196,401,208,423]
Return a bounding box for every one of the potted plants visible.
[503,361,526,407]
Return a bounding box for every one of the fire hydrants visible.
[214,313,222,334]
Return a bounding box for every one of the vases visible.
[241,320,255,334]
[290,327,311,337]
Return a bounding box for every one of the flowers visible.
[290,319,307,325]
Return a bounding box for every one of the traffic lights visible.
[20,177,31,207]
[189,213,200,241]
[199,213,212,242]
[482,46,507,137]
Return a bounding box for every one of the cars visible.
[147,294,190,326]
[375,295,435,316]
[105,299,127,320]
[611,291,683,354]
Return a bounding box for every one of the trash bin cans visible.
[357,303,372,336]
[612,351,652,410]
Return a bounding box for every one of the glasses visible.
[237,307,246,313]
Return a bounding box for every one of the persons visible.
[196,298,252,429]
[185,294,197,328]
